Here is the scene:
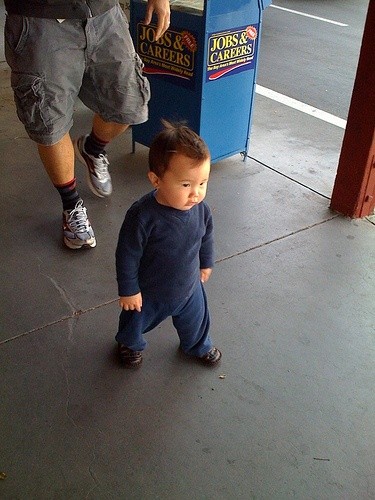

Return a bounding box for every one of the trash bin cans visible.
[128,0,273,163]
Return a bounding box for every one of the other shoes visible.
[118,341,143,368]
[203,346,223,365]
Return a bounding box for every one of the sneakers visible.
[62,200,97,251]
[75,135,111,198]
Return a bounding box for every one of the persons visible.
[113,126,222,369]
[4,0,171,250]
